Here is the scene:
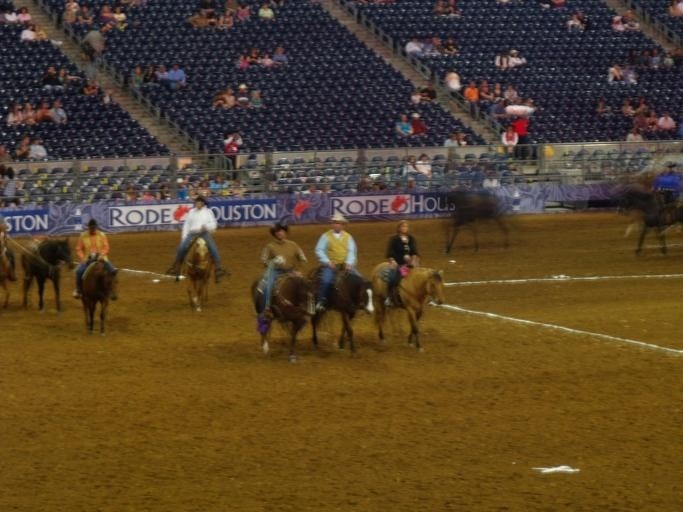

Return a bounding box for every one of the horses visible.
[440,190,511,255]
[369,260,447,353]
[616,188,683,258]
[21,234,79,315]
[0,224,18,309]
[181,235,216,314]
[245,270,319,365]
[77,260,118,339]
[307,262,377,359]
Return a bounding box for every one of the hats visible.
[327,208,348,223]
[87,219,97,228]
[196,195,208,204]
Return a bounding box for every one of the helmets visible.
[270,222,287,235]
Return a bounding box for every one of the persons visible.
[164,194,233,284]
[649,160,683,229]
[382,219,422,306]
[258,221,309,320]
[72,218,119,301]
[0,215,18,282]
[1,1,683,207]
[312,211,364,314]
